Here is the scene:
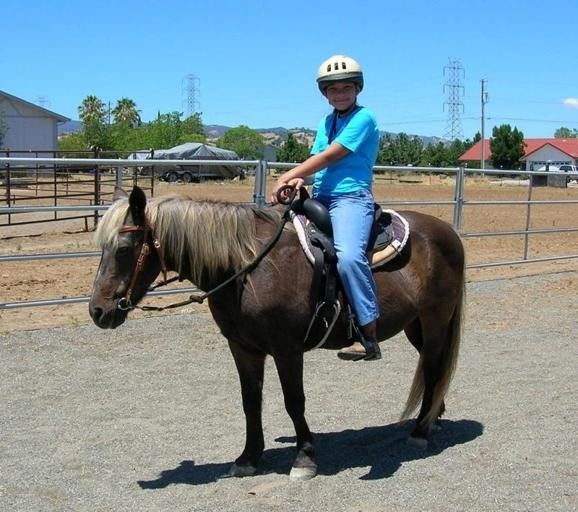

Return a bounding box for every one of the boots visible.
[338,320,381,360]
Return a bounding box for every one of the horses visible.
[88,184,467,482]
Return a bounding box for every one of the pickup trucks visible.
[128,142,242,182]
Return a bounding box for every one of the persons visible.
[269,55,383,361]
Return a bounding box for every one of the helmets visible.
[316,55,363,99]
